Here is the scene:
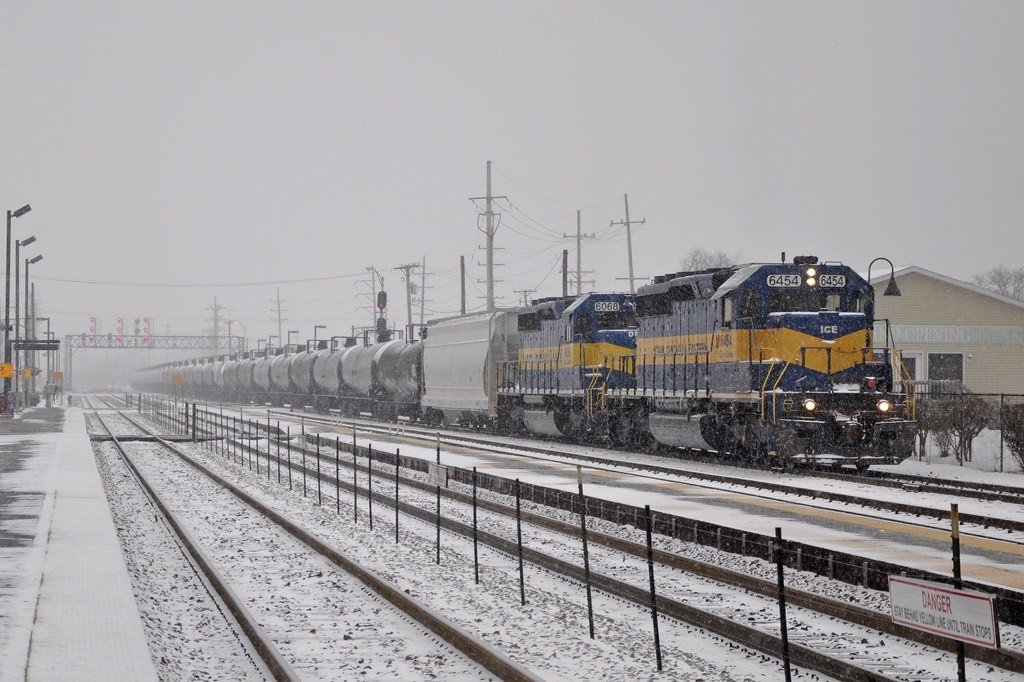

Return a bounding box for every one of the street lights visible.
[257,339,268,358]
[44,332,55,401]
[15,235,36,410]
[288,330,299,352]
[268,335,279,355]
[313,325,326,350]
[3,203,31,408]
[35,316,53,407]
[21,254,43,409]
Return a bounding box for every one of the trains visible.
[128,252,921,473]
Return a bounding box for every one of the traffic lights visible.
[376,289,387,310]
